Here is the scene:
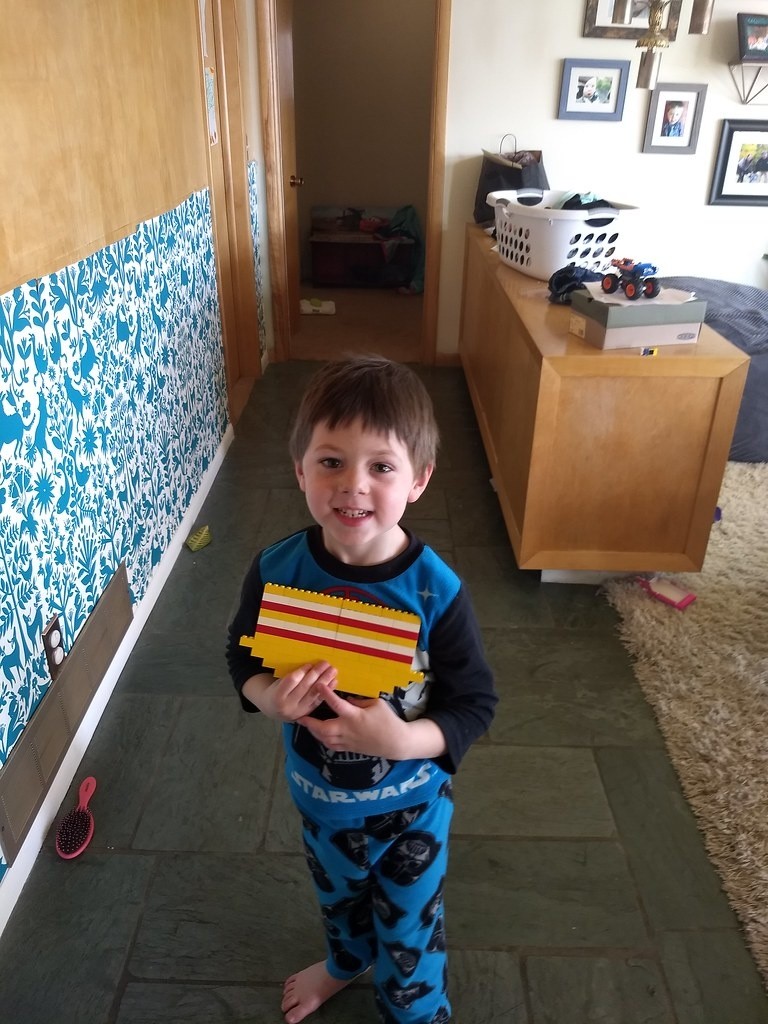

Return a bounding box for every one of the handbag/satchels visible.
[474,133,550,227]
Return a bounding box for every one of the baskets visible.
[485,185,618,279]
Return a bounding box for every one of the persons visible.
[576,75,600,104]
[737,148,768,183]
[226,354,498,1024]
[662,101,685,137]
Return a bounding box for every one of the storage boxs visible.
[308,230,420,287]
[486,188,642,282]
[569,290,709,350]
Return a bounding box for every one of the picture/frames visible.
[737,13,768,60]
[708,118,768,206]
[642,83,708,154]
[582,0,683,41]
[557,58,631,121]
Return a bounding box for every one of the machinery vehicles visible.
[601,257,661,301]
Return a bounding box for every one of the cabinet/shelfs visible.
[455,221,752,584]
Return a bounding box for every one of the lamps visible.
[611,0,715,90]
